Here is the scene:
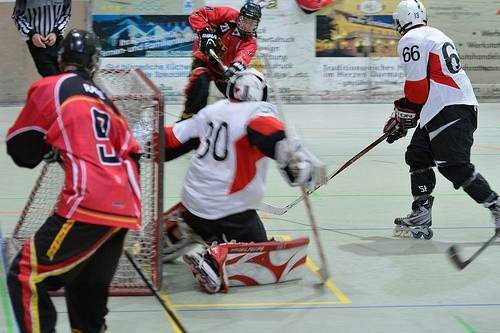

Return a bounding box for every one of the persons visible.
[180,2,263,120]
[383,0,500,236]
[12,0,72,78]
[5,29,142,333]
[136,67,329,294]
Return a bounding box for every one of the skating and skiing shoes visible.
[485,196,500,233]
[393,196,434,240]
[183,252,223,293]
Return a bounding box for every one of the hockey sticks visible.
[255,122,400,215]
[449,229,500,271]
[208,47,238,84]
[262,52,328,286]
[59,156,188,333]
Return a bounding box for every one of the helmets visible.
[236,3,261,36]
[393,0,428,36]
[225,71,268,103]
[57,28,100,81]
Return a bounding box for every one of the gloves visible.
[223,62,245,83]
[383,98,419,145]
[197,29,218,58]
